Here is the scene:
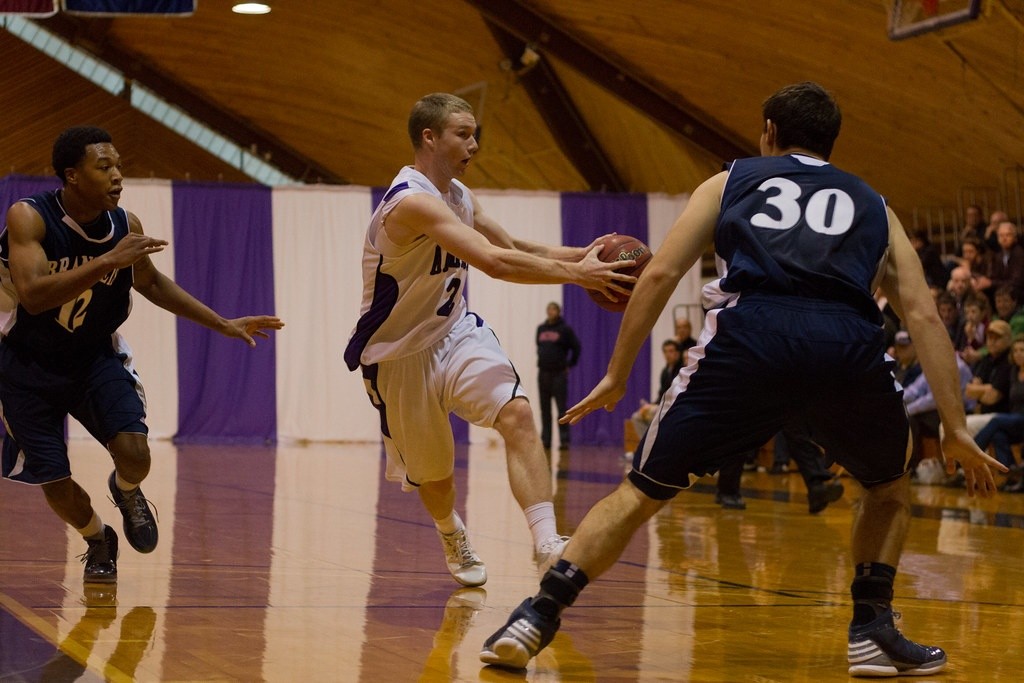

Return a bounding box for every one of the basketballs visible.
[585,234,654,313]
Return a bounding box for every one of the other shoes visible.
[715,494,746,509]
[559,442,568,450]
[807,483,844,514]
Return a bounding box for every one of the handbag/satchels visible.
[916,456,948,486]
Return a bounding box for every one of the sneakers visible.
[743,455,758,470]
[946,468,966,487]
[435,509,488,587]
[769,461,790,474]
[533,533,572,584]
[835,466,851,477]
[846,611,948,677]
[76,524,119,582]
[107,468,160,554]
[1004,474,1022,492]
[480,597,562,669]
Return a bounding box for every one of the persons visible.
[536,299,582,449]
[347,93,640,586]
[630,205,1023,514]
[0,126,284,581]
[479,83,1007,675]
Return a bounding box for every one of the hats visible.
[893,330,912,348]
[986,319,1013,337]
